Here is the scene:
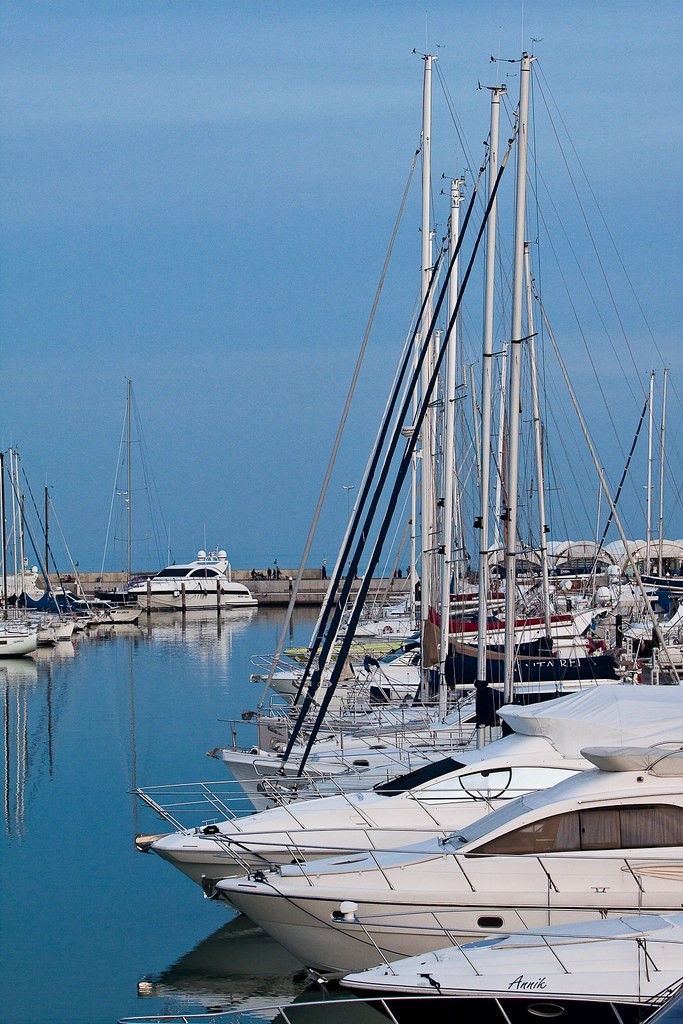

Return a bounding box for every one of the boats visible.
[126,548,258,611]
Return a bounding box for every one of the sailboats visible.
[135,34,682,1024]
[0,446,143,656]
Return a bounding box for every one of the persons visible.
[321,565,328,580]
[398,568,403,579]
[406,566,411,579]
[249,566,283,580]
